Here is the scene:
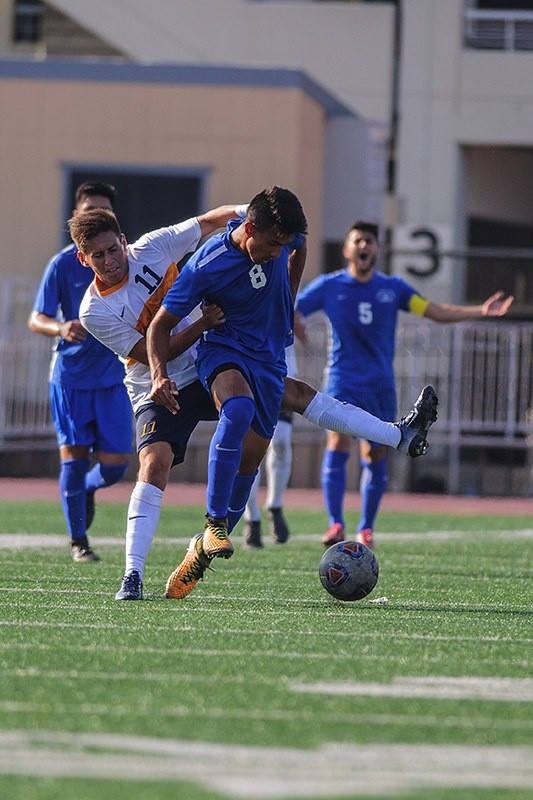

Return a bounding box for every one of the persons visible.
[244,346,298,549]
[292,221,515,549]
[146,186,307,597]
[26,181,134,561]
[66,203,438,601]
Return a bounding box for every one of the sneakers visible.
[85,490,94,529]
[397,385,438,458]
[116,571,143,601]
[72,538,101,562]
[357,528,375,549]
[323,522,345,544]
[202,512,234,559]
[266,512,289,542]
[244,521,263,547]
[166,534,218,599]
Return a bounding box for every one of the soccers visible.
[318,540,378,601]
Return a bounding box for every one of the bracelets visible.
[235,204,250,217]
